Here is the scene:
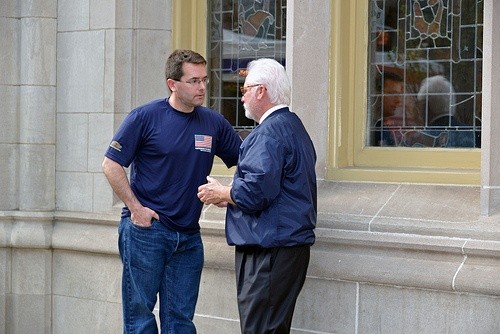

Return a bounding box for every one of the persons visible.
[198,59,318,334]
[373,74,473,144]
[102,49,247,334]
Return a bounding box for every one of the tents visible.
[221,30,287,81]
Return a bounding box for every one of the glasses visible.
[240,84,267,93]
[167,76,208,87]
